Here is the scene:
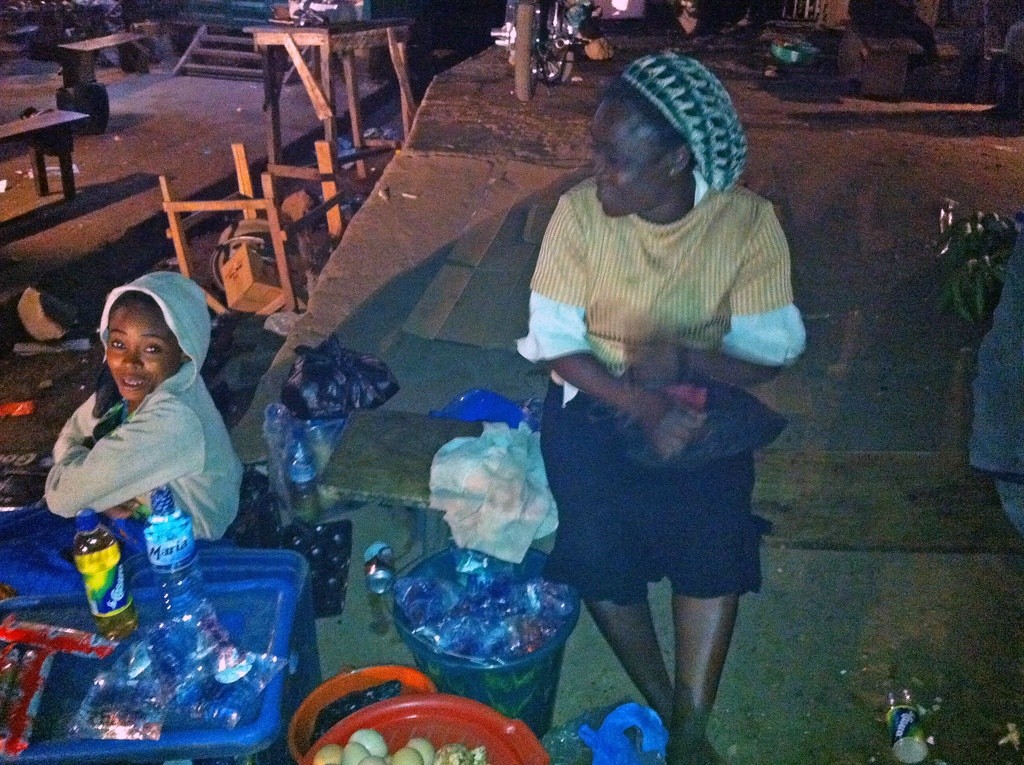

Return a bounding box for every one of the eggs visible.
[311,728,435,765]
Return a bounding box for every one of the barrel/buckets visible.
[384,543,582,740]
[297,697,551,765]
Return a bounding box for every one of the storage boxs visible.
[0,548,313,765]
[221,245,286,320]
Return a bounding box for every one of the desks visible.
[243,24,412,193]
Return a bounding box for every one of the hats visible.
[619,51,749,195]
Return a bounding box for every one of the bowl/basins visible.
[770,40,818,66]
[286,664,438,764]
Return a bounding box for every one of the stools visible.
[838,20,925,101]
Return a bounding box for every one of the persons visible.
[514,48,807,765]
[44,272,249,590]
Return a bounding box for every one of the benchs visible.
[0,112,91,199]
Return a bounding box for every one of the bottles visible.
[885,682,929,764]
[142,486,209,619]
[283,420,322,525]
[73,509,139,640]
